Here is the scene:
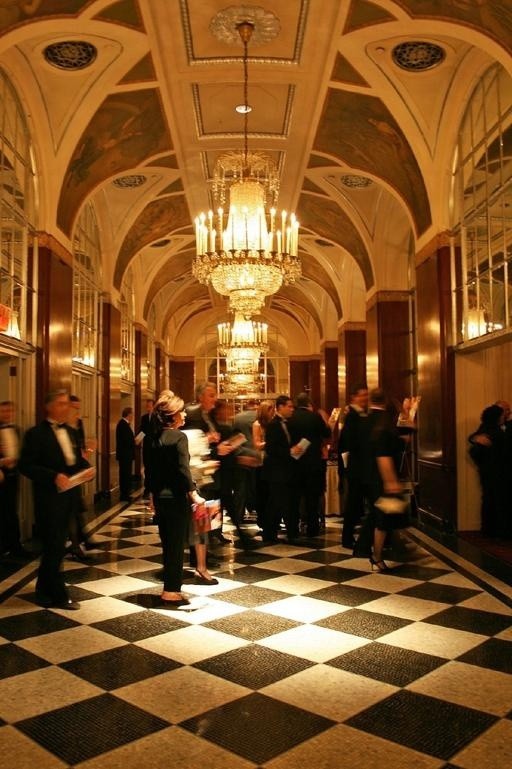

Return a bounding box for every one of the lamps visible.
[461,229,489,344]
[192,20,303,321]
[213,312,273,402]
[1,230,21,340]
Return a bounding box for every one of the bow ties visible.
[55,423,66,429]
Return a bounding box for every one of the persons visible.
[116,383,413,610]
[468,400,511,553]
[2,389,96,610]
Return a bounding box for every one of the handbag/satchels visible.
[373,494,410,530]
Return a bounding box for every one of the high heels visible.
[370,557,389,571]
[195,569,218,585]
